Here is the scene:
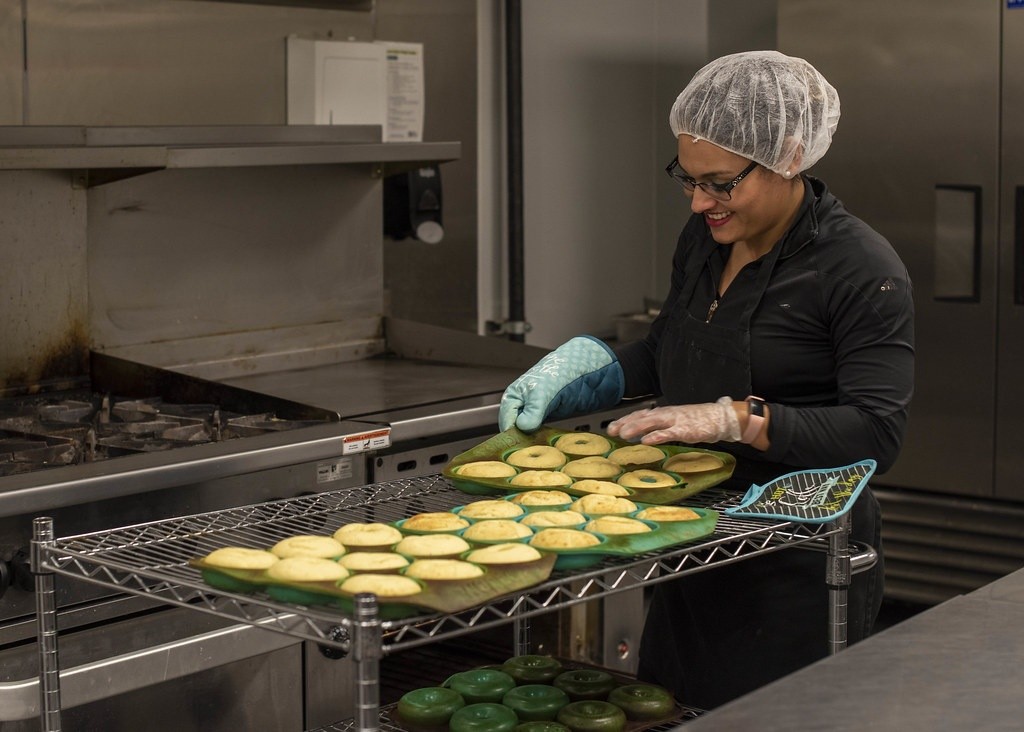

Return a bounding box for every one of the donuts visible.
[458,432,724,491]
[397,655,673,732]
[202,488,701,596]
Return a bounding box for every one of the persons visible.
[494,48,917,732]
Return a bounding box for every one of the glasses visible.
[665,155,759,201]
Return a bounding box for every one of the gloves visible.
[498,334,624,435]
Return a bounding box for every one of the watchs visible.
[738,395,766,444]
